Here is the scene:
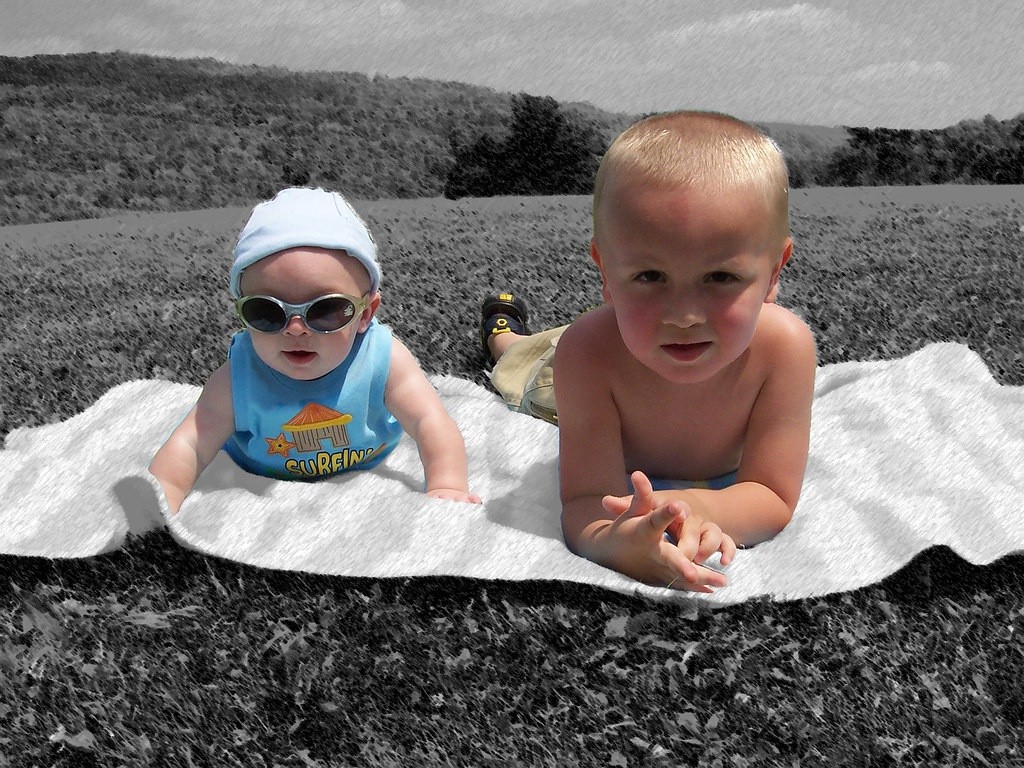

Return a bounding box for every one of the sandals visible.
[481,294,533,372]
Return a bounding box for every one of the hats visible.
[228,186,381,300]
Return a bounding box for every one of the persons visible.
[147,186,483,517]
[483,109,817,592]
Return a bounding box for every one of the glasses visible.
[229,292,371,333]
